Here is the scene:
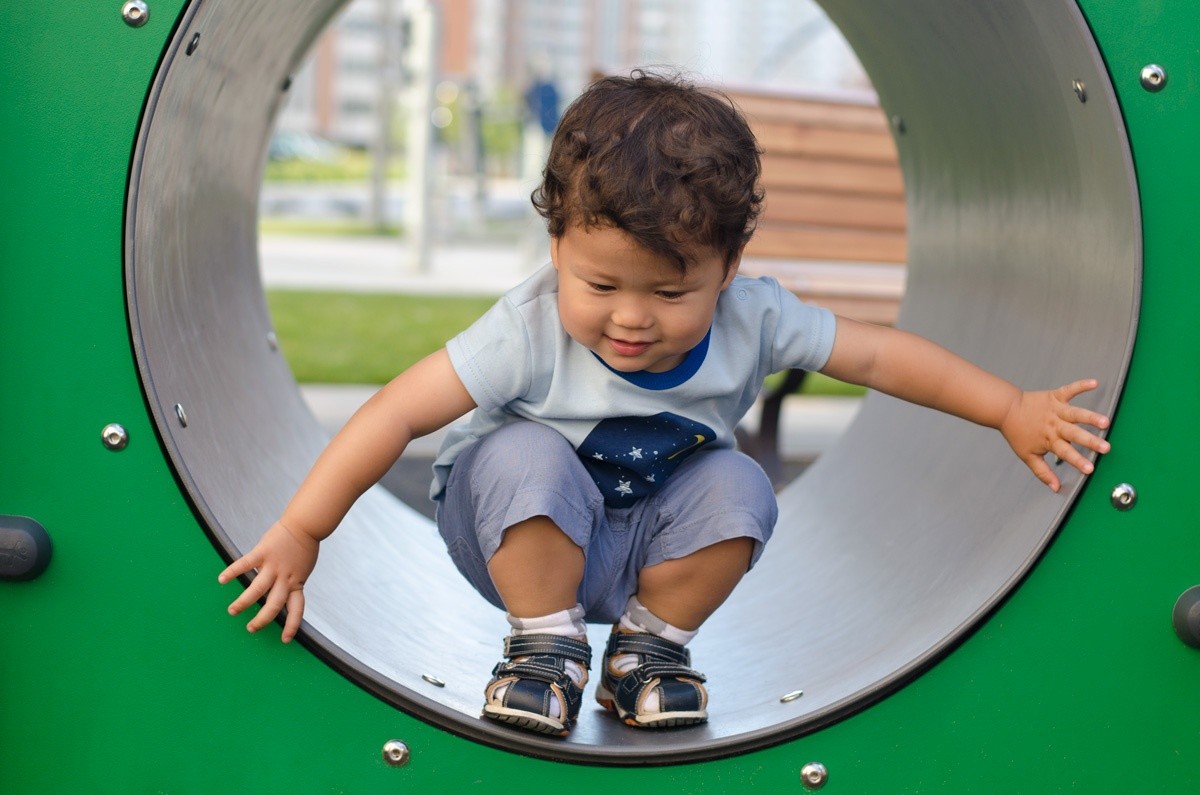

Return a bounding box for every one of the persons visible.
[217,68,1110,739]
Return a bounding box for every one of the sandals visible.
[481,633,592,737]
[595,622,709,730]
